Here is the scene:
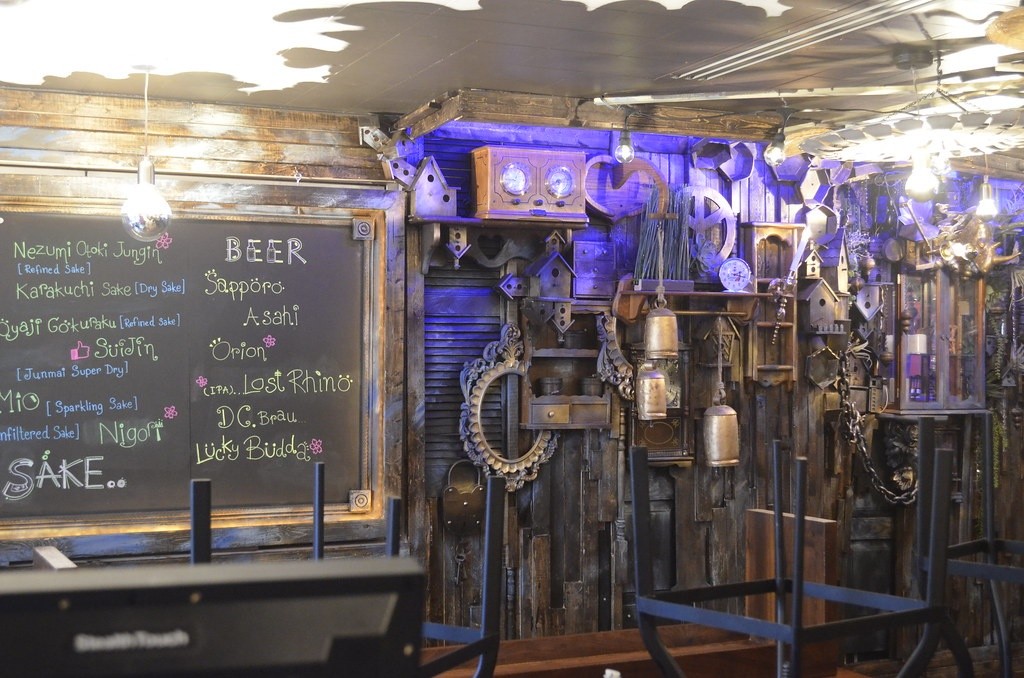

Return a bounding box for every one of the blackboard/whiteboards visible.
[0,193,387,544]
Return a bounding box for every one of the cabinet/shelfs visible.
[572,240,616,301]
[740,221,808,392]
[894,239,987,409]
[521,306,621,429]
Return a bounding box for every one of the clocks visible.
[629,339,689,457]
[718,258,749,293]
[468,146,590,223]
